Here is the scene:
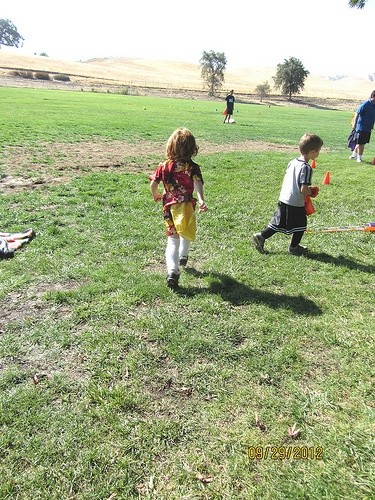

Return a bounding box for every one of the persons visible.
[348,90,375,162]
[223,90,236,123]
[254,132,323,255]
[150,126,207,286]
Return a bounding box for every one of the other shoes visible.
[180,258,188,265]
[253,233,265,254]
[290,244,307,253]
[168,272,179,285]
[349,155,355,160]
[357,159,362,162]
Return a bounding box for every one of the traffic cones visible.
[305,197,315,216]
[310,159,316,168]
[322,171,330,185]
[223,109,227,115]
